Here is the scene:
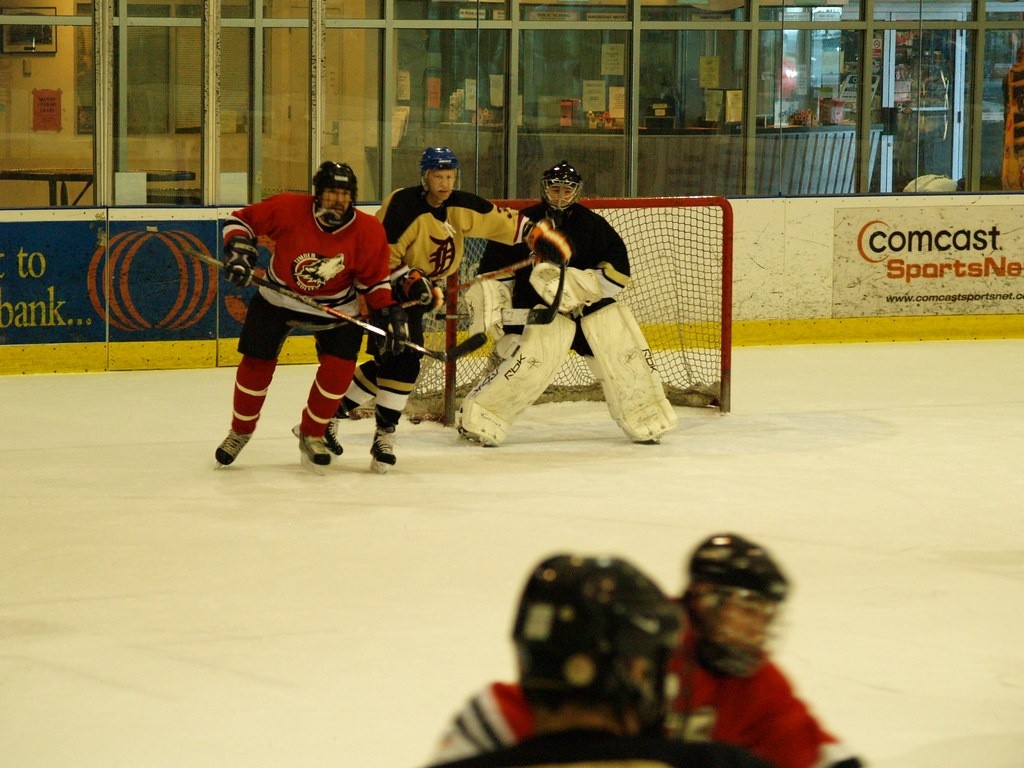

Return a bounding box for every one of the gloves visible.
[400,267,445,313]
[521,218,573,267]
[372,303,412,356]
[221,236,260,289]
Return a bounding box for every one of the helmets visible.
[312,160,358,204]
[417,145,461,171]
[509,550,684,694]
[685,533,792,604]
[539,161,583,191]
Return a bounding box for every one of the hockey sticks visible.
[431,260,568,326]
[179,246,487,363]
[287,256,540,335]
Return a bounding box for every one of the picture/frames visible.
[0,6,57,53]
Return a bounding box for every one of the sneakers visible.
[292,417,344,460]
[298,432,333,476]
[369,424,396,474]
[213,427,258,471]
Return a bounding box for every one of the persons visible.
[419,531,865,768]
[215,160,407,476]
[458,167,678,448]
[292,147,572,474]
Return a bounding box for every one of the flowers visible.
[793,106,813,124]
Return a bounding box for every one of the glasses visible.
[715,585,781,628]
[621,630,673,669]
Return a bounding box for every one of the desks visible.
[147,188,202,206]
[0,169,197,207]
